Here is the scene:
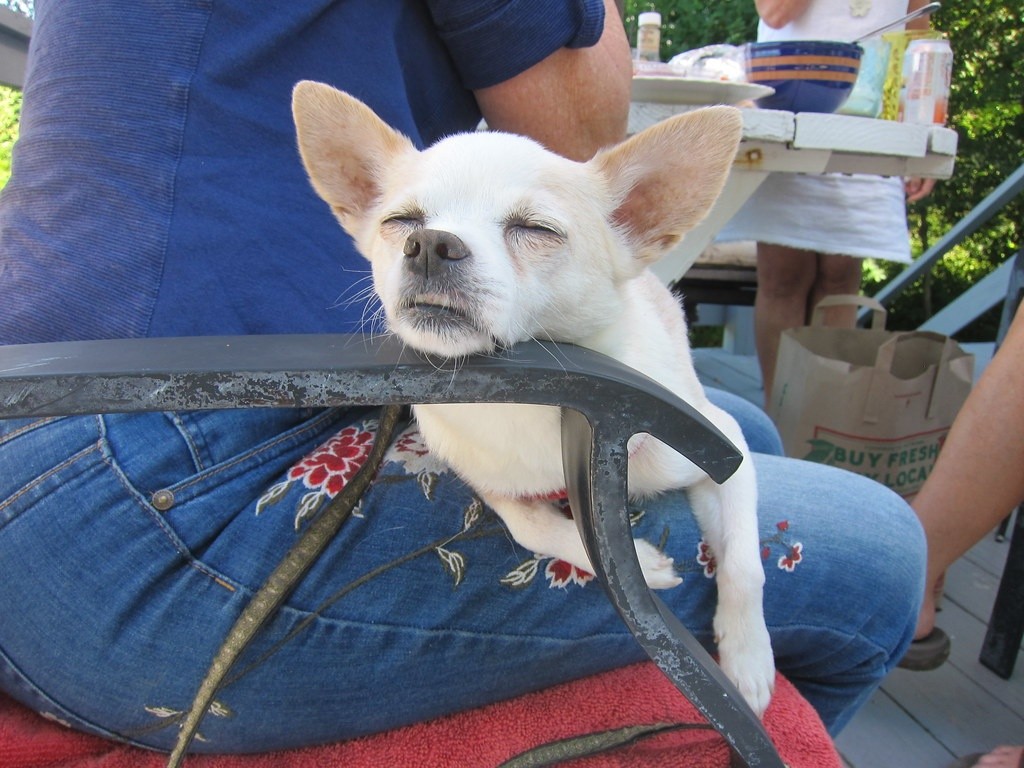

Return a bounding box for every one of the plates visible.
[630,73,777,104]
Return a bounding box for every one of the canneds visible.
[898,38,953,129]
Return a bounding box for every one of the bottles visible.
[637,12,662,62]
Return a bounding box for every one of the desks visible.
[627,97,957,293]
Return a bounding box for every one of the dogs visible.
[292,78,775,724]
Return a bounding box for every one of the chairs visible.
[0,333,840,768]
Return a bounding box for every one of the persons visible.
[753,0,938,413]
[895,295,1024,768]
[1,0,929,767]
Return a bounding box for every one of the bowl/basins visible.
[747,41,863,111]
